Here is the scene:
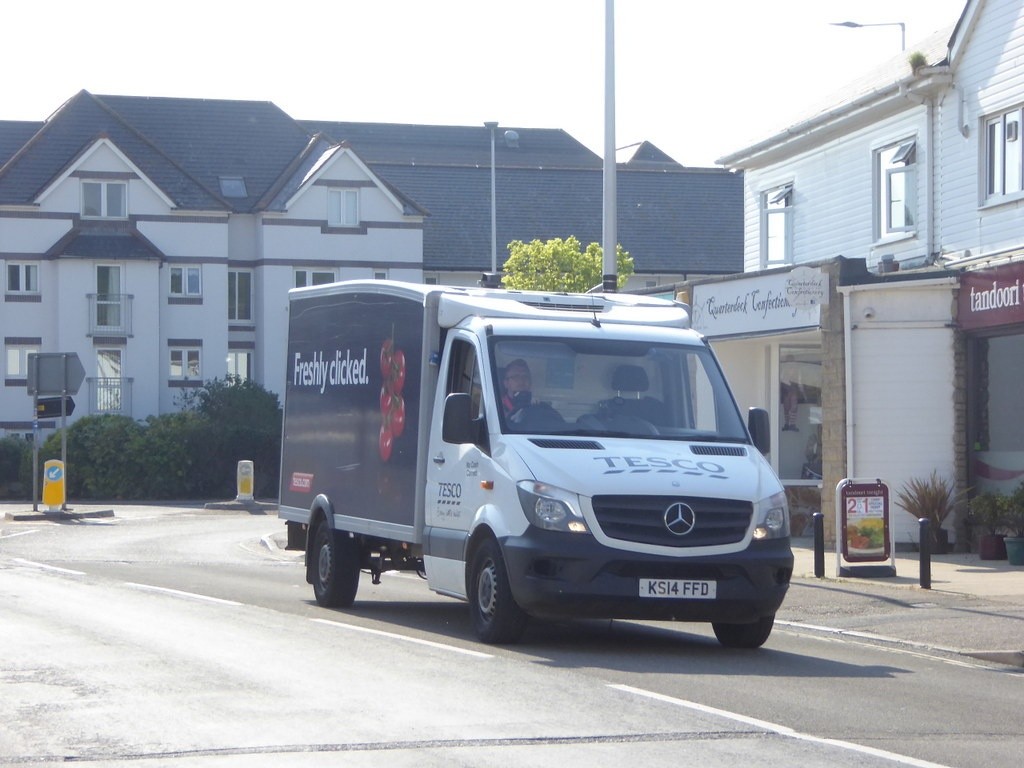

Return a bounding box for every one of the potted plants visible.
[894,467,977,555]
[996,480,1024,566]
[966,491,1014,560]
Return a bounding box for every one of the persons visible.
[501,359,531,417]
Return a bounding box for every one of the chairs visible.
[596,364,668,426]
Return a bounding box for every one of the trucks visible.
[276,278,795,649]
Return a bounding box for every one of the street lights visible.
[483,120,500,274]
